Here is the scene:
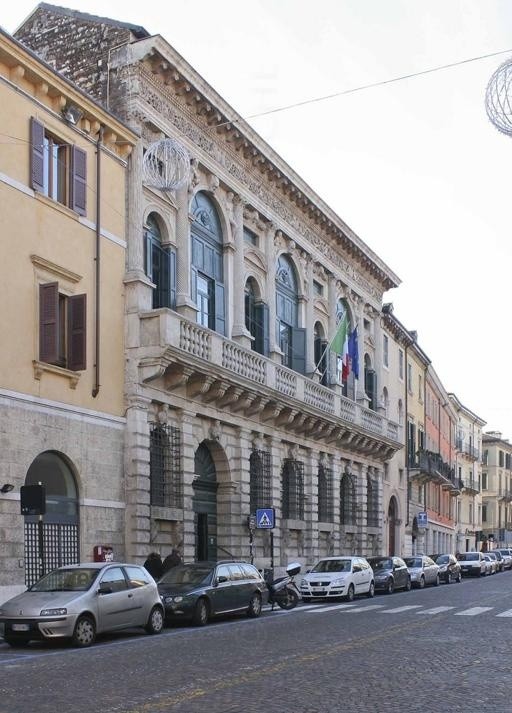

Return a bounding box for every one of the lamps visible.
[0,484,14,493]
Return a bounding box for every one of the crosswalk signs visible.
[257,508,274,528]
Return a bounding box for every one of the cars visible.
[299,548,512,602]
[0,561,165,647]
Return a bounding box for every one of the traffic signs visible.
[418,511,428,526]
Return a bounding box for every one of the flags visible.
[326,311,348,368]
[346,327,360,381]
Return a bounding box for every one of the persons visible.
[143,552,165,583]
[162,548,182,580]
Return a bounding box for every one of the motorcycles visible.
[257,562,302,611]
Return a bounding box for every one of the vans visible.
[155,559,266,626]
